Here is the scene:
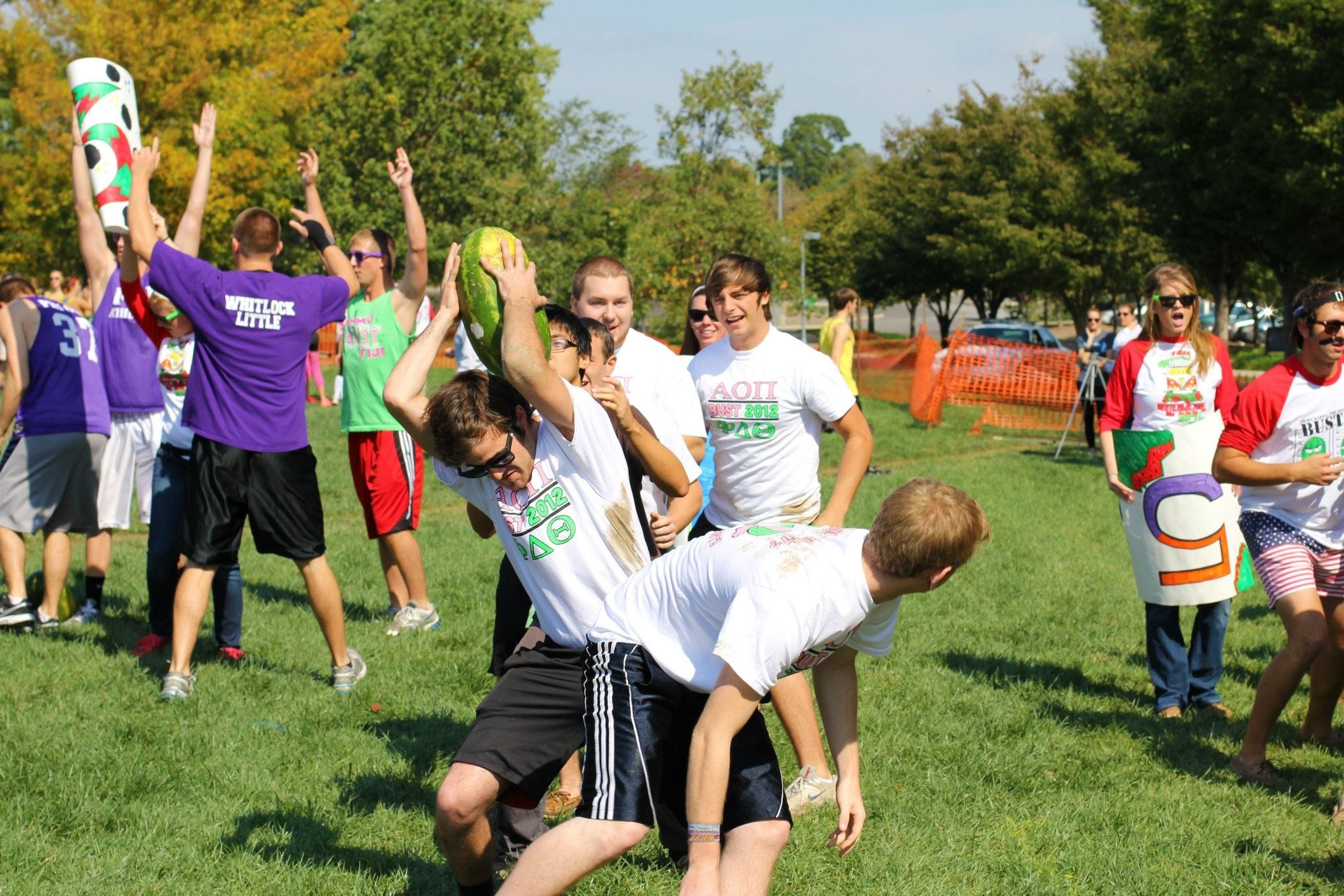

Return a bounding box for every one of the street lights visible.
[800,232,822,345]
[778,160,793,241]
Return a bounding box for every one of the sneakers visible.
[542,789,582,819]
[784,766,838,815]
[0,594,60,631]
[159,671,197,702]
[330,648,366,697]
[370,599,441,637]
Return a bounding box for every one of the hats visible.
[1286,298,1309,348]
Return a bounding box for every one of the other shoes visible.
[1158,706,1182,719]
[1205,703,1233,718]
[216,646,244,660]
[1230,756,1280,786]
[60,598,103,625]
[130,632,172,660]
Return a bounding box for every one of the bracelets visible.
[686,821,721,833]
[687,831,722,841]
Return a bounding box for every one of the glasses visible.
[1117,313,1128,316]
[1314,319,1344,335]
[347,251,383,263]
[549,339,577,351]
[1159,294,1197,308]
[1087,319,1099,322]
[456,428,516,479]
[688,309,718,322]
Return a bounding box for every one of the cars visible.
[1200,302,1284,345]
[933,320,1075,388]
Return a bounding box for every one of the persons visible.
[1075,265,1344,788]
[1,103,993,896]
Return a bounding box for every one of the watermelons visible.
[457,226,550,372]
